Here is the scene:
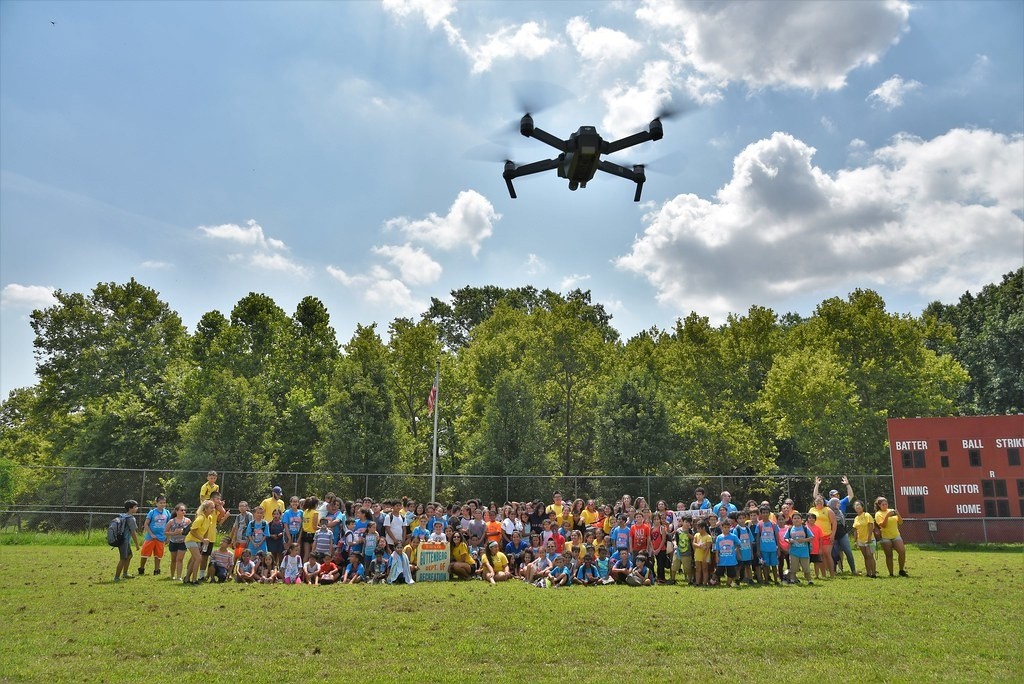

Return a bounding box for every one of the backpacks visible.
[181,513,211,535]
[334,532,354,565]
[833,519,847,538]
[871,519,883,542]
[108,516,133,547]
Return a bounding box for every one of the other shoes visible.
[808,580,813,585]
[899,570,908,577]
[192,580,200,585]
[789,579,800,583]
[866,574,876,579]
[123,574,135,578]
[138,568,145,574]
[154,569,160,575]
[889,574,894,578]
[170,576,174,581]
[199,577,208,583]
[837,569,844,576]
[655,577,785,588]
[210,579,215,584]
[852,571,860,577]
[178,577,183,581]
[183,576,191,585]
[114,577,120,582]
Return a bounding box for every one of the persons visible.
[813,476,862,576]
[199,470,227,524]
[230,485,862,587]
[852,500,877,578]
[182,499,215,585]
[138,494,172,577]
[205,534,234,584]
[195,491,226,582]
[113,499,141,581]
[164,502,193,582]
[874,496,909,578]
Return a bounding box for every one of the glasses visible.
[452,536,460,538]
[553,496,562,498]
[833,494,840,497]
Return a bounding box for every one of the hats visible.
[829,490,840,496]
[273,486,283,496]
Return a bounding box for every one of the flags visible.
[428,375,438,411]
